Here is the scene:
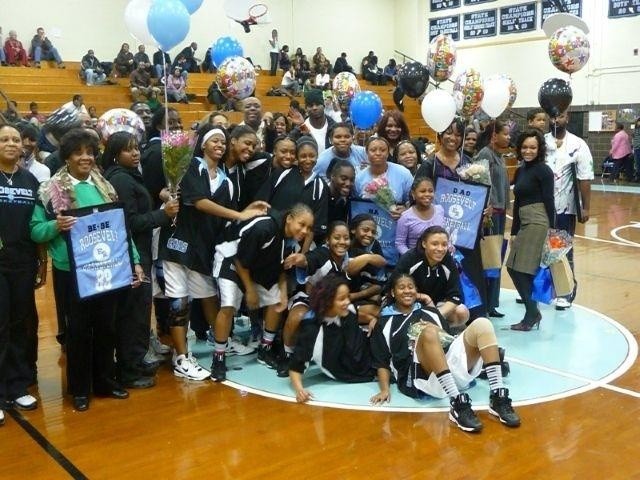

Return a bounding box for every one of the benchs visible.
[0,58,520,188]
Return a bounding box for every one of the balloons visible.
[124,0,204,50]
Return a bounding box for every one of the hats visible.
[304,90,324,107]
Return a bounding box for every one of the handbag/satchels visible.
[550,255,574,297]
[531,268,556,303]
[480,215,508,279]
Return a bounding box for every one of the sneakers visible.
[73,336,211,410]
[448,393,482,431]
[194,329,295,385]
[0,394,37,425]
[488,389,517,425]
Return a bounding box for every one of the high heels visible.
[511,313,541,331]
